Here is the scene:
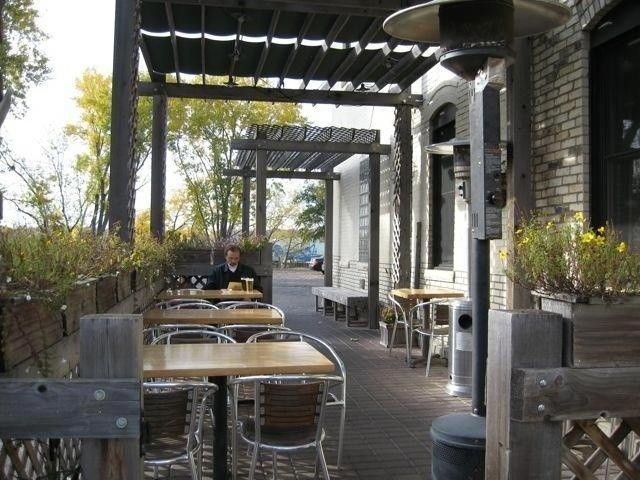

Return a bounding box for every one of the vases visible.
[530,290,640,369]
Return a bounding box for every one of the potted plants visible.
[379,304,420,348]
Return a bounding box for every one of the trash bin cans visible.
[430,413,486,479]
[445,298,473,397]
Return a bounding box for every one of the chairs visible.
[388,288,469,378]
[143,288,347,480]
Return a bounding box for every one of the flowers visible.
[497,198,640,297]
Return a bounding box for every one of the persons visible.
[206,245,264,309]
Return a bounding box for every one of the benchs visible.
[312,287,368,327]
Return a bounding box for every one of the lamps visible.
[380,0,572,88]
[422,138,510,181]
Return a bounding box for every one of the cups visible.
[241,278,254,293]
